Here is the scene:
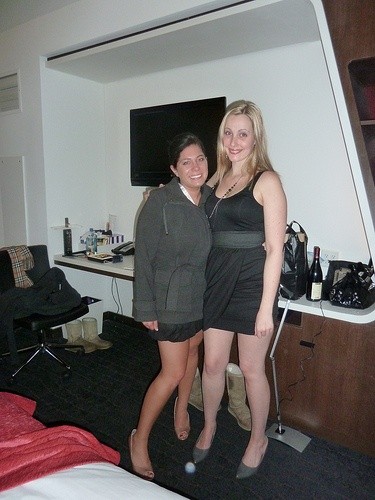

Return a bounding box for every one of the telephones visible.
[111,241,135,256]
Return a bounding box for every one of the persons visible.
[128,133,213,481]
[145,100,287,480]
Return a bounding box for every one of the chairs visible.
[0,245,89,385]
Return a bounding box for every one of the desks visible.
[54,252,375,457]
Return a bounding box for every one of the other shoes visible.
[193,426,216,464]
[174,396,190,441]
[128,429,154,481]
[236,436,268,478]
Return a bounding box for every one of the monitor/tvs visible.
[130,97,227,187]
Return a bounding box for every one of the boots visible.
[65,320,95,352]
[82,317,112,349]
[188,367,221,412]
[225,362,252,430]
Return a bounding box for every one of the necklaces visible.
[222,175,243,198]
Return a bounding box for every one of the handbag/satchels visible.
[280,221,309,300]
[330,262,375,310]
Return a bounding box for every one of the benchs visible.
[0,392,189,500]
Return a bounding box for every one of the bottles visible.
[86,227,97,254]
[306,246,323,303]
